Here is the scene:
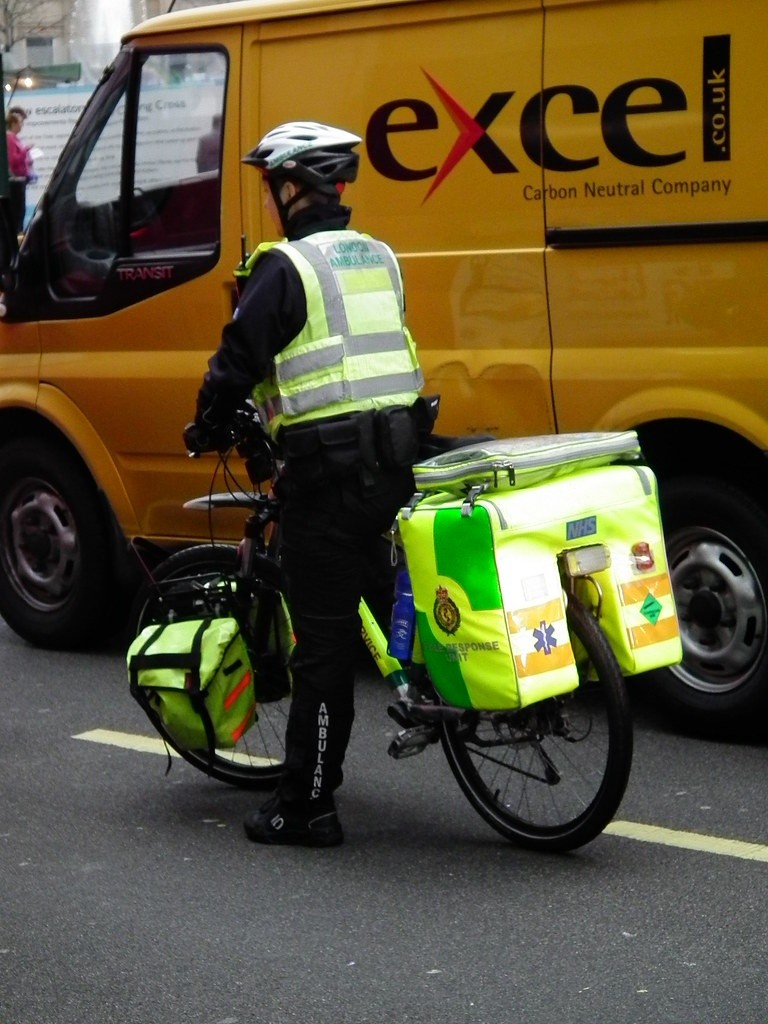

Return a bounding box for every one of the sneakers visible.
[245,796,343,849]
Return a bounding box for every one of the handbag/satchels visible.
[204,579,300,690]
[125,627,255,750]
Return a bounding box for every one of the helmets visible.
[238,121,362,194]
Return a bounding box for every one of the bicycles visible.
[125,404,634,857]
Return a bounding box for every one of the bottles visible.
[387,568,415,660]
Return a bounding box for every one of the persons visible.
[6,109,35,232]
[195,113,223,171]
[196,121,427,847]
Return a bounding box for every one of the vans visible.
[1,0,768,744]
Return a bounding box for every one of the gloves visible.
[182,423,221,453]
